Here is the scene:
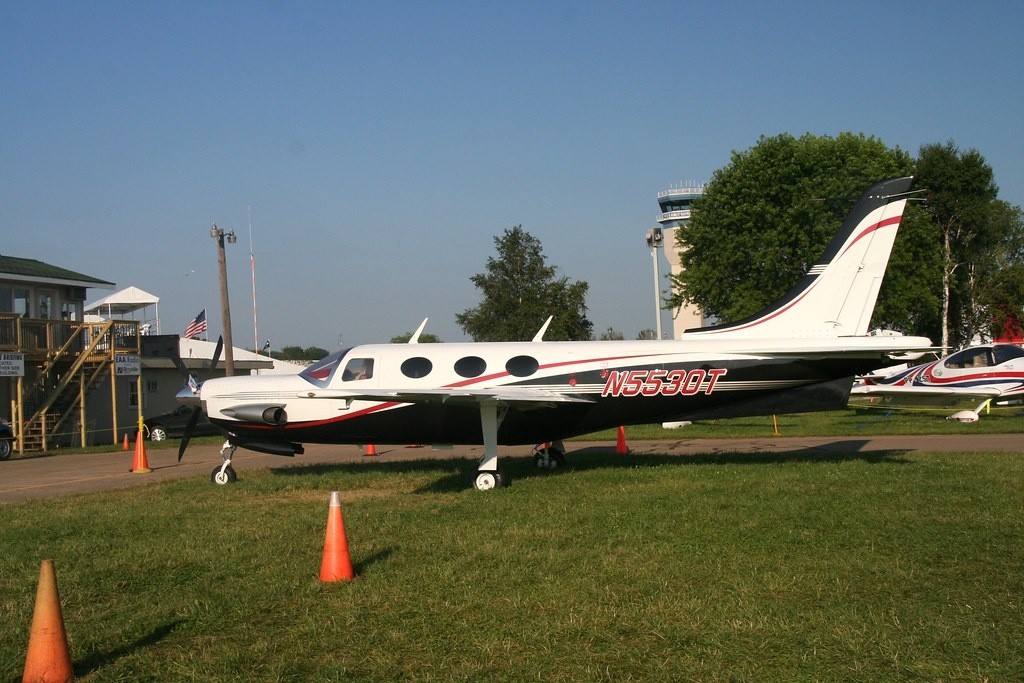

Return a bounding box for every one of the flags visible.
[263,341,269,352]
[184,309,206,339]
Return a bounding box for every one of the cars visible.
[0,423,17,462]
[133,404,222,442]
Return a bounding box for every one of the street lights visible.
[645,227,664,340]
[210,220,240,376]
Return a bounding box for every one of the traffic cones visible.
[404,444,424,448]
[122,434,131,451]
[129,431,151,471]
[18,559,77,683]
[318,490,356,582]
[616,426,627,454]
[361,444,378,457]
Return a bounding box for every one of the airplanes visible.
[164,173,952,494]
[846,341,1024,422]
